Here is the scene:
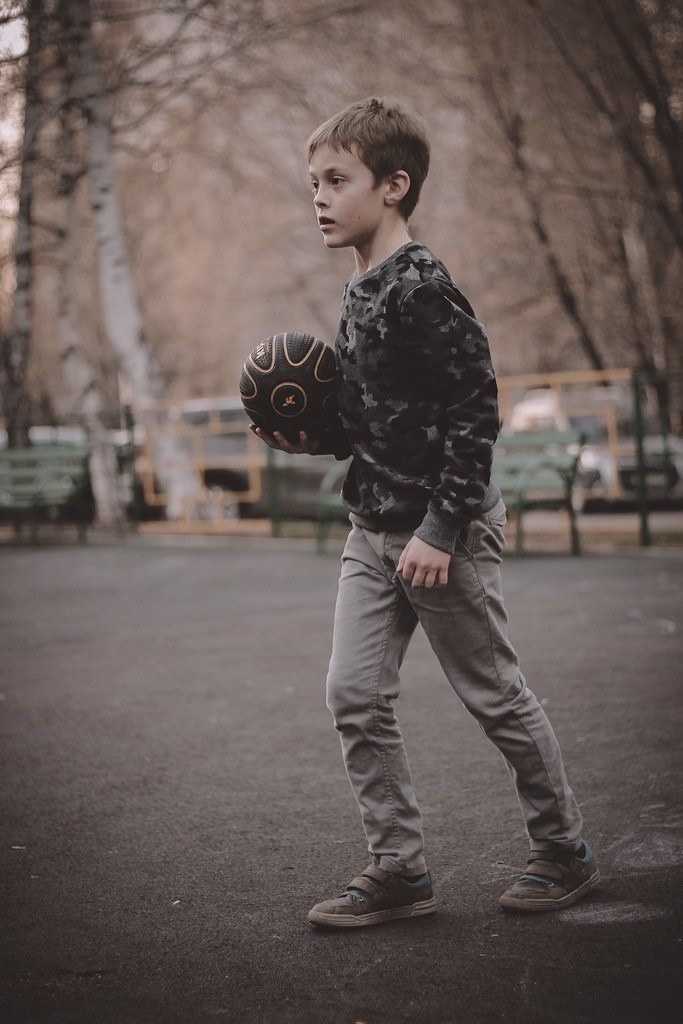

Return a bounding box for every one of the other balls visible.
[238,331,335,442]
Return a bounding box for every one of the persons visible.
[248,98,604,926]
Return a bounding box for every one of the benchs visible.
[0,445,93,546]
[316,432,585,558]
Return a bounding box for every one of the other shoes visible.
[308,863,437,927]
[500,839,601,912]
